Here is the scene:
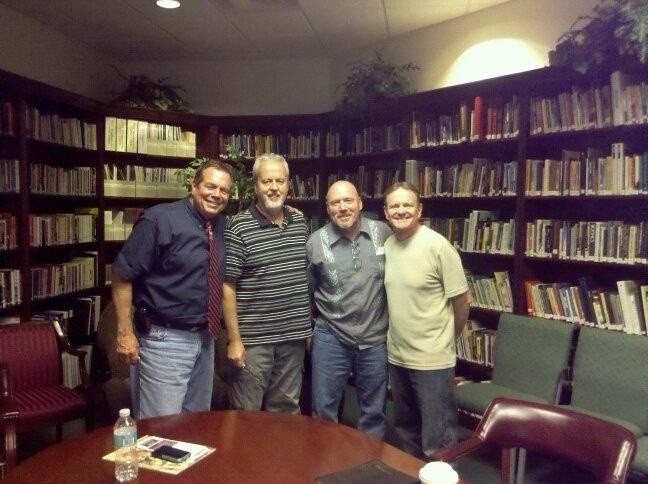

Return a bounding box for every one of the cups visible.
[416,461,460,484]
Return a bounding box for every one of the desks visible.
[1,412,469,484]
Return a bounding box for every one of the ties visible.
[204,219,223,341]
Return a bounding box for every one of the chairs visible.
[627,435,646,480]
[2,318,92,473]
[517,323,647,482]
[451,310,577,482]
[426,399,638,483]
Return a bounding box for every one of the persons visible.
[111,155,237,424]
[304,175,394,444]
[382,180,473,459]
[220,149,314,414]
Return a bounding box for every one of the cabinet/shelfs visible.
[0,61,104,398]
[324,61,647,403]
[105,116,197,292]
[214,109,325,242]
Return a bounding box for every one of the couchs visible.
[93,298,230,413]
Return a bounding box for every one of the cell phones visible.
[151,444,192,463]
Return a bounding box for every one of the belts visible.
[146,317,211,334]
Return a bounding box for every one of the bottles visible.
[113,407,138,481]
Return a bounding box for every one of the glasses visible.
[350,241,361,270]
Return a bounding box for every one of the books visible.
[105,116,196,287]
[2,101,102,389]
[404,92,519,385]
[100,432,218,476]
[220,122,401,201]
[525,71,648,336]
